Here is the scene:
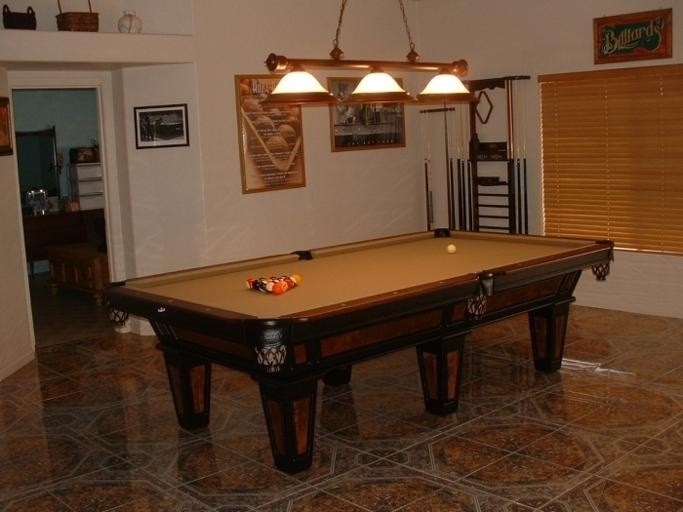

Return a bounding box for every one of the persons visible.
[141,113,167,141]
[336,90,401,146]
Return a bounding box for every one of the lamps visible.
[258,0,480,105]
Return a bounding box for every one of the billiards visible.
[447,244,456,253]
[246,275,300,295]
[241,93,300,159]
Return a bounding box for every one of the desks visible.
[48,244,108,307]
[102,227,614,474]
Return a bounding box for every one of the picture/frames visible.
[593,7,672,64]
[0,96,13,157]
[133,74,405,195]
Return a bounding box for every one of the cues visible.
[424,110,435,231]
[448,83,472,231]
[504,79,529,236]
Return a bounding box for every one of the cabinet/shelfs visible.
[69,163,105,211]
[23,210,104,278]
